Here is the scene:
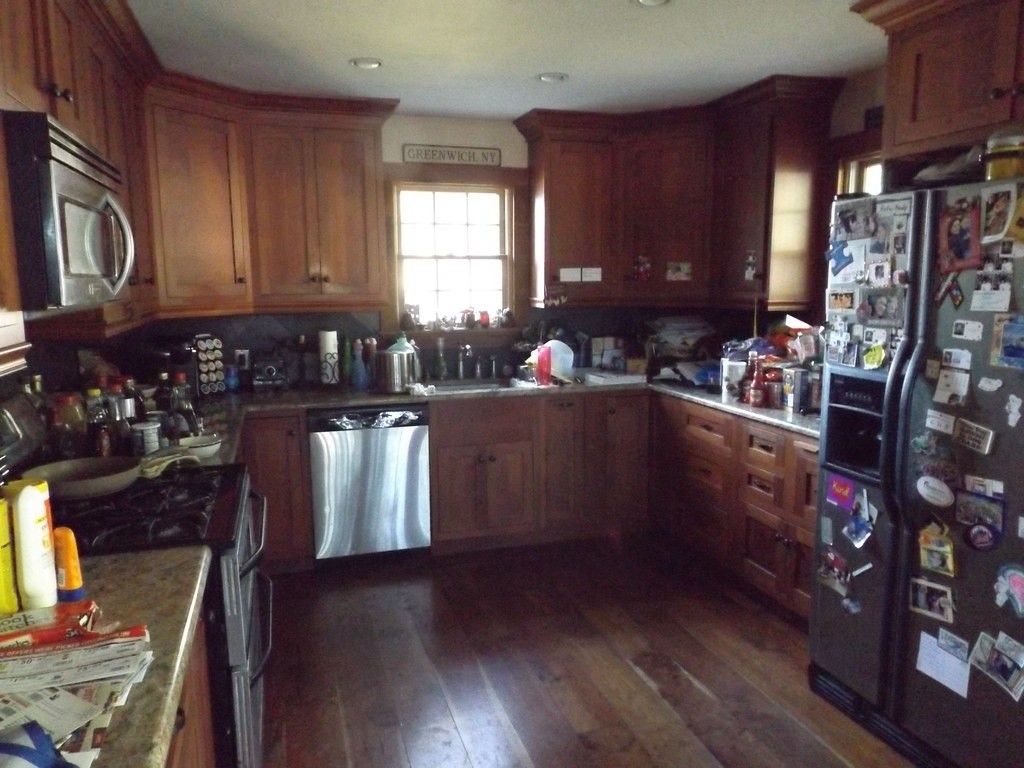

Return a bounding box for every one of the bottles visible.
[224,330,422,394]
[464,309,514,327]
[742,351,782,409]
[0,479,83,619]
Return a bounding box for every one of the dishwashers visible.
[305,405,433,578]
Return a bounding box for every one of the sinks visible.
[424,375,519,392]
[21,454,143,498]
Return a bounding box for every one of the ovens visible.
[206,464,273,768]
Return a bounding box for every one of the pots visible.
[19,447,199,502]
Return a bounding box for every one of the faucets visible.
[0,408,25,440]
[457,343,473,380]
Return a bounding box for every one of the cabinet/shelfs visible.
[850,1,1024,163]
[0,0,401,379]
[513,72,848,312]
[427,390,821,624]
[242,409,314,574]
[163,601,217,768]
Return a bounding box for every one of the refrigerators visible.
[808,185,1024,768]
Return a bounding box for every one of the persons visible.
[984,194,1007,236]
[930,552,944,569]
[871,295,887,320]
[869,224,889,254]
[947,218,973,260]
[912,577,928,610]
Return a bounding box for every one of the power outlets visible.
[234,349,249,370]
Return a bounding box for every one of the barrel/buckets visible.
[978,126,1024,182]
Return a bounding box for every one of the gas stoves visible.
[0,394,251,549]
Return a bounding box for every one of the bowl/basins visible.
[179,436,222,458]
[515,339,575,386]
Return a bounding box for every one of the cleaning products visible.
[0,476,86,615]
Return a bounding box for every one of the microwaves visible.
[5,109,135,326]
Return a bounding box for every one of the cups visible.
[719,357,747,403]
[535,346,550,387]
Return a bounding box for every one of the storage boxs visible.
[783,368,809,414]
